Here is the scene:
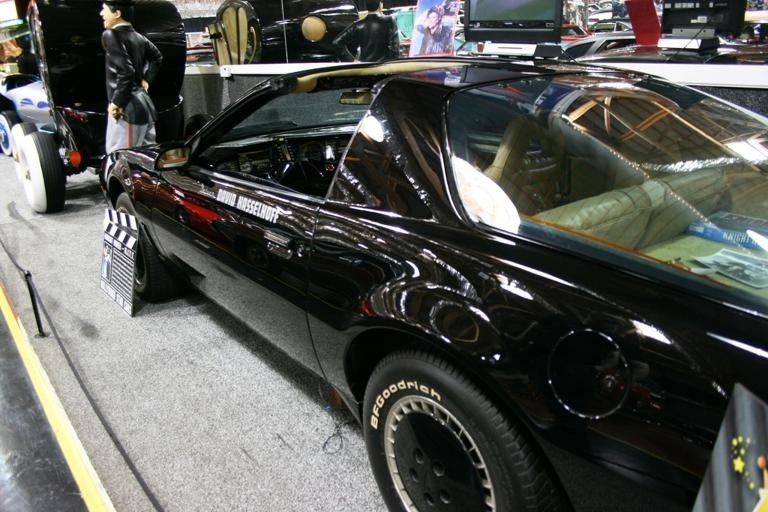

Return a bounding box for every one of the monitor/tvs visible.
[660,0,748,39]
[463,0,563,43]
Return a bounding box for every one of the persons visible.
[414,5,453,57]
[331,0,400,62]
[98,0,164,155]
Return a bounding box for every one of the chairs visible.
[482,116,727,250]
